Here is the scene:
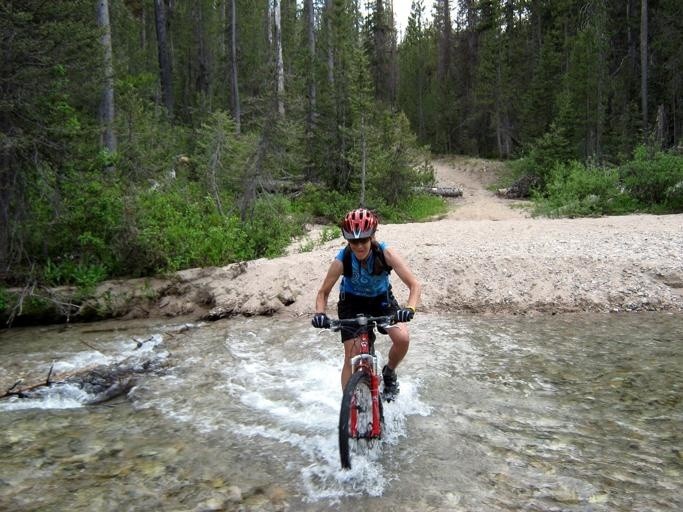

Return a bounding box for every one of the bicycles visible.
[328,314,413,469]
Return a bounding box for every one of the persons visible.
[311,208,422,440]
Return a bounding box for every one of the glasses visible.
[347,237,372,244]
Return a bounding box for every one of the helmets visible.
[341,208,378,240]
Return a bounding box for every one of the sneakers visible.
[382,365,399,400]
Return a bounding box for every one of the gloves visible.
[311,313,331,329]
[395,308,415,323]
